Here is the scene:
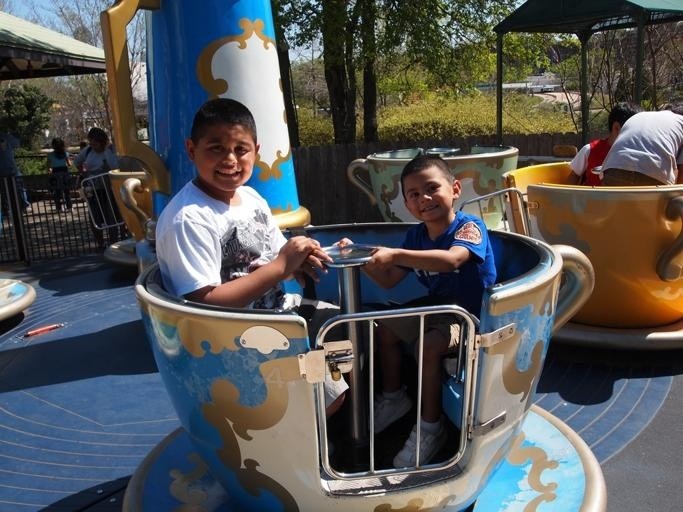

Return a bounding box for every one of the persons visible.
[331,155,497,469]
[80,141,86,150]
[0,125,33,213]
[155,99,350,458]
[47,138,78,217]
[565,101,683,186]
[74,127,118,256]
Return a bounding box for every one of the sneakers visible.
[394,422,447,469]
[374,389,413,436]
